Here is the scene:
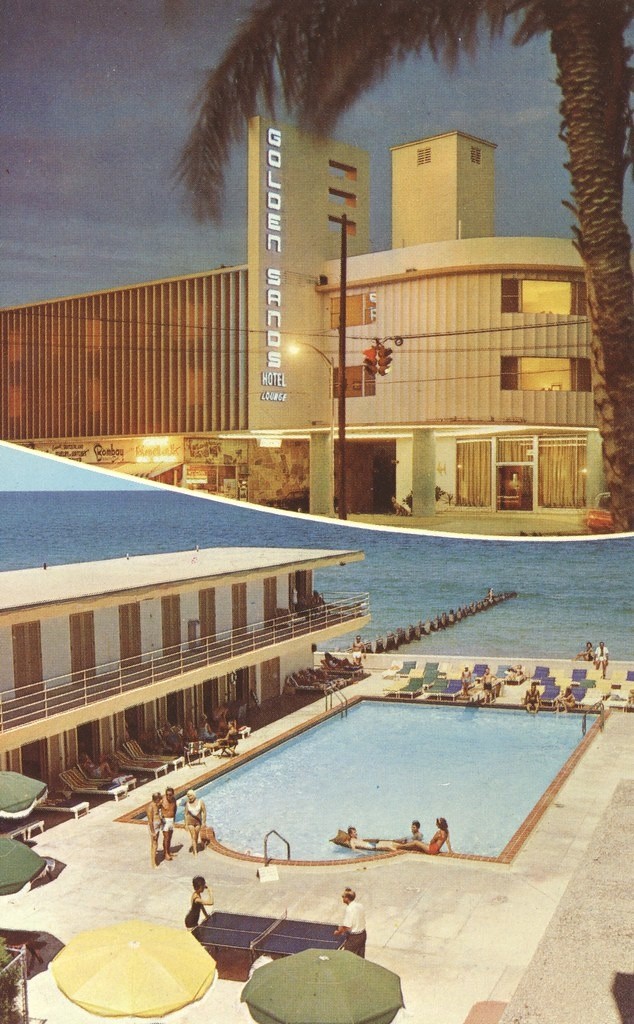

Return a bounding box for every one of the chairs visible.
[381,660,634,712]
[287,658,364,693]
[5,713,251,842]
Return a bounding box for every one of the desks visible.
[186,748,207,768]
[192,909,353,958]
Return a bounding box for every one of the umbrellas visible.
[0,770,48,833]
[51,921,217,1024]
[240,948,405,1024]
[0,837,47,897]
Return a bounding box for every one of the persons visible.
[571,642,596,661]
[325,636,367,670]
[146,792,162,870]
[332,886,367,959]
[184,875,214,932]
[184,789,207,859]
[461,664,576,714]
[161,787,177,860]
[347,817,454,855]
[593,642,609,679]
[78,703,238,780]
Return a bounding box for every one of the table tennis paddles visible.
[205,884,208,889]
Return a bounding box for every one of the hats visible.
[436,817,449,829]
